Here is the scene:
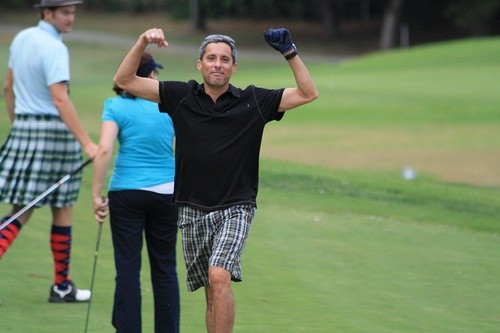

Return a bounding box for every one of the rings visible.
[150,32,155,36]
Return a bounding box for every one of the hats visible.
[34,0,84,8]
[137,59,163,76]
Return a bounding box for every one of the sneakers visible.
[48,281,92,302]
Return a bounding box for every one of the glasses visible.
[204,34,236,46]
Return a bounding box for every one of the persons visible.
[112,26,319,333]
[0,0,107,303]
[92,50,189,333]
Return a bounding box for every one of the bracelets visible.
[284,51,297,60]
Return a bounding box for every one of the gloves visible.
[263,27,295,54]
